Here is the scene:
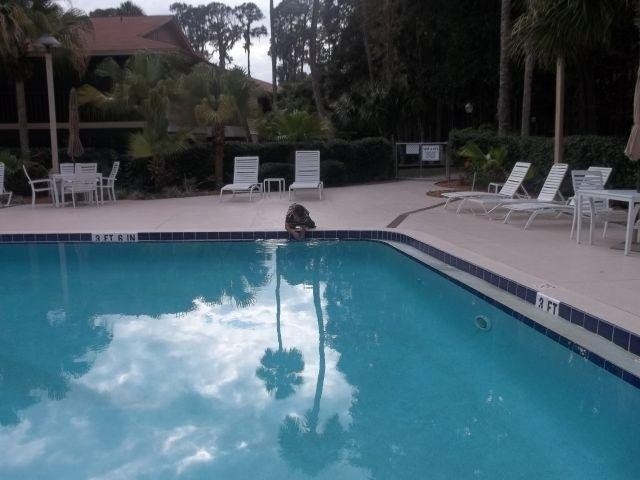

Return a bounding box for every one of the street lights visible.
[463,101,474,129]
[30,33,64,176]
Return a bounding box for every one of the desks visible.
[47,173,104,208]
[264,178,285,200]
[577,189,640,256]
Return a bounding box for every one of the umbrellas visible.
[67,86,85,173]
[624,64,640,193]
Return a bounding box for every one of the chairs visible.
[95,161,120,204]
[219,156,262,201]
[570,170,627,246]
[1,162,12,207]
[59,164,75,202]
[289,150,323,202]
[62,162,99,209]
[23,165,55,207]
[441,162,530,215]
[502,167,612,231]
[456,163,569,220]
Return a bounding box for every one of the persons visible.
[285,203,315,240]
